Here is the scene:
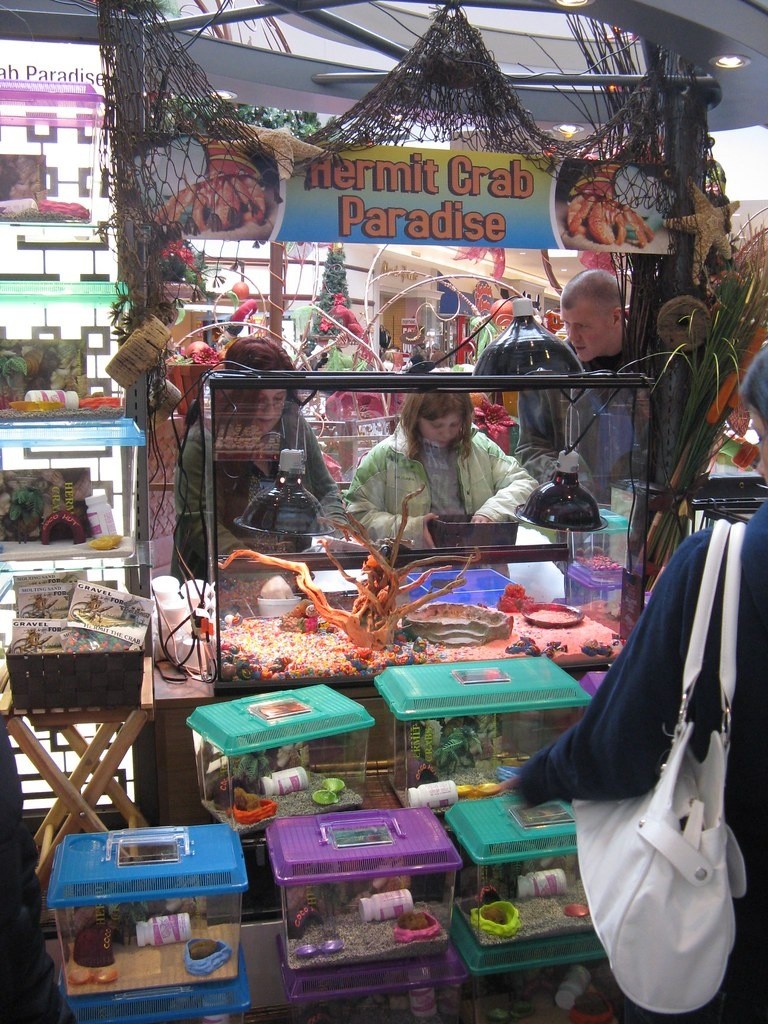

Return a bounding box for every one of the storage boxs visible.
[0,77,105,225]
[265,805,464,968]
[273,933,471,1023]
[375,654,595,817]
[408,569,519,607]
[0,415,147,561]
[48,821,248,1000]
[57,933,251,1023]
[4,639,146,709]
[185,681,376,837]
[565,561,623,632]
[444,790,602,945]
[571,507,633,576]
[200,372,656,695]
[427,513,519,548]
[447,901,631,1024]
[1,278,134,418]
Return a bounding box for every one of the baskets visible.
[6,643,145,709]
[431,514,519,547]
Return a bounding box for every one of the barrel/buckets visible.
[258,596,302,617]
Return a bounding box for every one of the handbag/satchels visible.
[571,519,748,1015]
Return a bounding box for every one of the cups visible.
[152,576,211,670]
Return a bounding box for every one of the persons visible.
[500,344,767,1023]
[517,269,650,504]
[172,336,353,584]
[347,387,538,550]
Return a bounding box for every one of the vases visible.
[167,363,224,415]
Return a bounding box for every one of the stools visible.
[0,654,155,882]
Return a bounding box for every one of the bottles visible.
[555,966,591,1010]
[86,496,116,539]
[261,766,309,796]
[359,889,413,921]
[136,913,191,946]
[517,869,568,898]
[409,987,436,1017]
[407,780,458,808]
[26,390,79,411]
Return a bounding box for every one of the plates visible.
[521,603,585,628]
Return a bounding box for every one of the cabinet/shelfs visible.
[155,657,610,826]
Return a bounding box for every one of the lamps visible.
[516,450,609,534]
[234,447,337,535]
[471,296,585,375]
